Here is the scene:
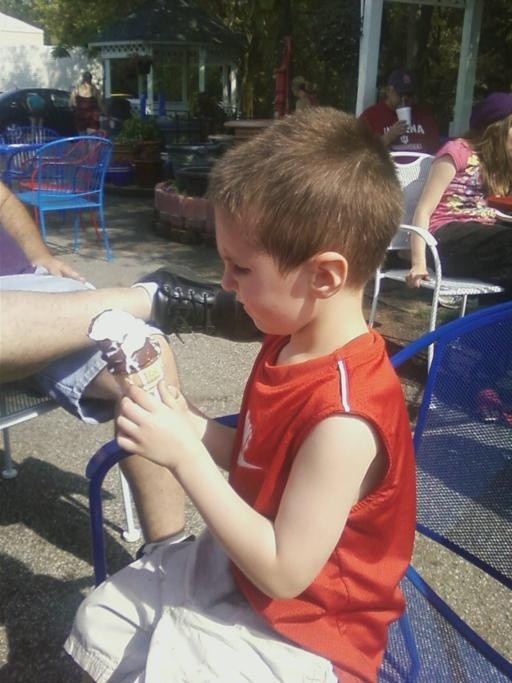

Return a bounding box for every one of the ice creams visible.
[87,309,163,405]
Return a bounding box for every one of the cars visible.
[0,86,131,143]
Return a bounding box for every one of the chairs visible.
[14,135,113,262]
[85,296,511,681]
[367,150,503,376]
[1,123,106,244]
[0,267,144,542]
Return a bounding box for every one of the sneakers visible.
[136,535,196,559]
[132,267,266,342]
[475,387,511,424]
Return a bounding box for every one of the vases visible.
[108,133,248,197]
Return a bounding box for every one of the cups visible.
[395,106,412,130]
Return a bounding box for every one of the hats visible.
[388,70,415,93]
[470,93,512,129]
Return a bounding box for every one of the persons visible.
[359,68,441,268]
[62,105,415,683]
[0,176,264,560]
[70,70,101,160]
[406,92,512,425]
[23,91,45,137]
[290,76,319,111]
